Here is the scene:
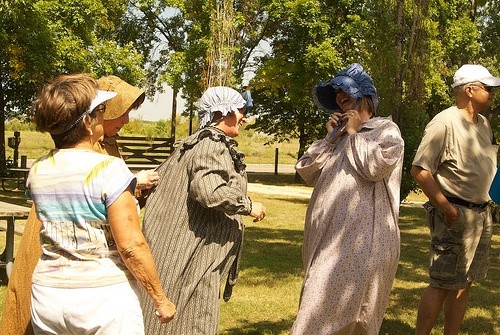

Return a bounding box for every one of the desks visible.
[0,201,31,269]
[6,168,31,192]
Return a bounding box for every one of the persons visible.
[94,75,159,199]
[25,75,176,335]
[291,62,404,335]
[137,86,266,334]
[411,65,500,335]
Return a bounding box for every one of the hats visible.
[96,75,145,120]
[313,63,376,139]
[199,86,247,129]
[452,64,500,88]
[49,89,117,134]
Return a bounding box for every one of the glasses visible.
[95,104,106,113]
[465,83,496,92]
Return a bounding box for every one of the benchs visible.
[0,220,26,235]
[1,177,26,182]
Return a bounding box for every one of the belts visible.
[444,195,492,209]
